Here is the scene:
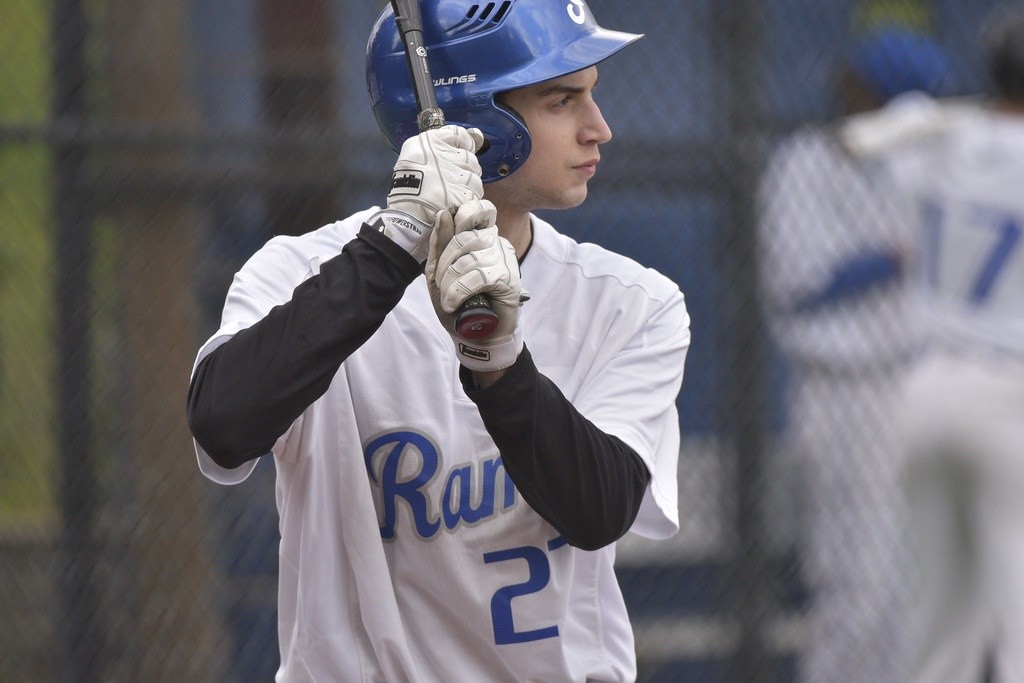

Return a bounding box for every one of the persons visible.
[187,0,691,683]
[754,22,1024,683]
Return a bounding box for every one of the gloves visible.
[364,123,485,267]
[424,198,525,374]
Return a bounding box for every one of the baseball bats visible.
[386,2,501,342]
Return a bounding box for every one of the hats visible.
[855,26,955,111]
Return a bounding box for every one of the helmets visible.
[365,0,646,184]
